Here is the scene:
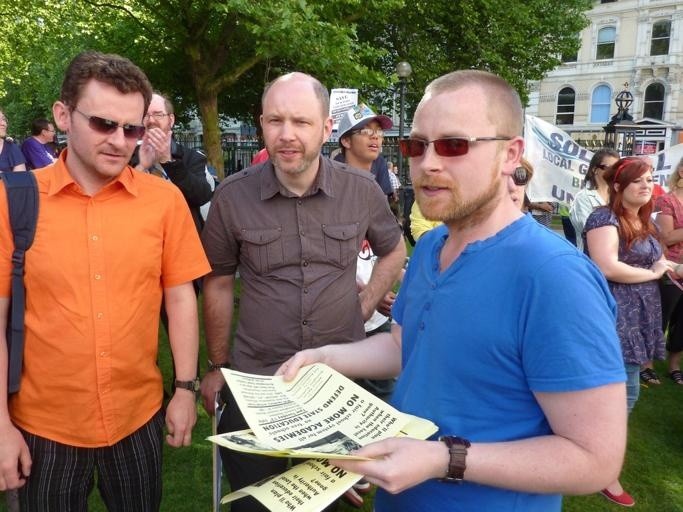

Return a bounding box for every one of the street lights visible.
[394,58,412,189]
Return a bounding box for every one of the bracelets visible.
[437,435,471,484]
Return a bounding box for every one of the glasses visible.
[596,164,611,171]
[396,136,515,157]
[67,104,146,139]
[0,117,8,123]
[41,128,55,135]
[511,167,530,186]
[343,129,384,138]
[143,114,171,120]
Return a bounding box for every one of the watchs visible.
[173,378,201,395]
[206,358,231,373]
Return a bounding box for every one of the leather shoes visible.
[596,482,636,507]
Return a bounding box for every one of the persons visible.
[273,68,629,512]
[198,71,408,511]
[506,147,683,508]
[1,91,221,334]
[0,49,215,512]
[329,102,417,508]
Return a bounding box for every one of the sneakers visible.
[341,487,364,507]
[352,479,371,493]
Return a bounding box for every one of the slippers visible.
[639,368,661,385]
[667,368,683,385]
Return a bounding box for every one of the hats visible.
[337,103,393,139]
